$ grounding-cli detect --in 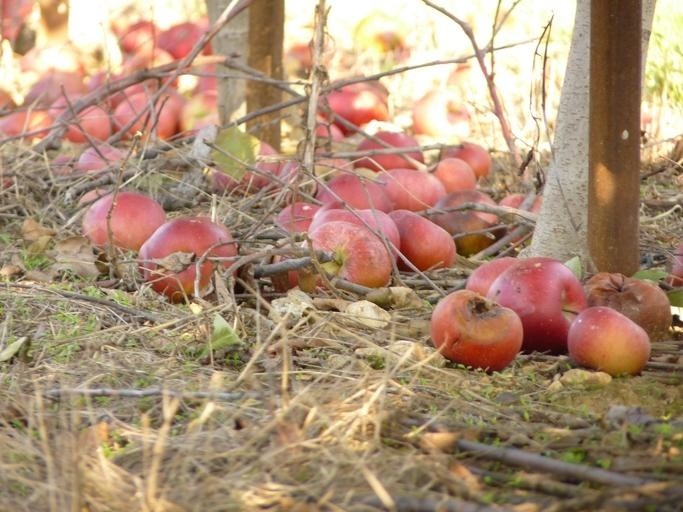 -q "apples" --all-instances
[1,1,673,378]
